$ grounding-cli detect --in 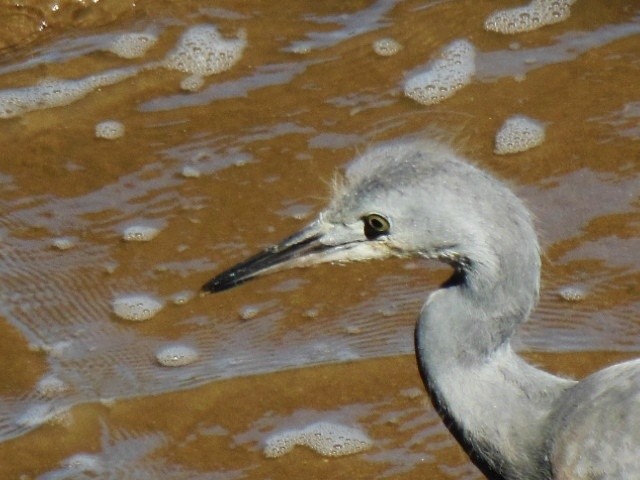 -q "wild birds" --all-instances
[197,133,640,480]
